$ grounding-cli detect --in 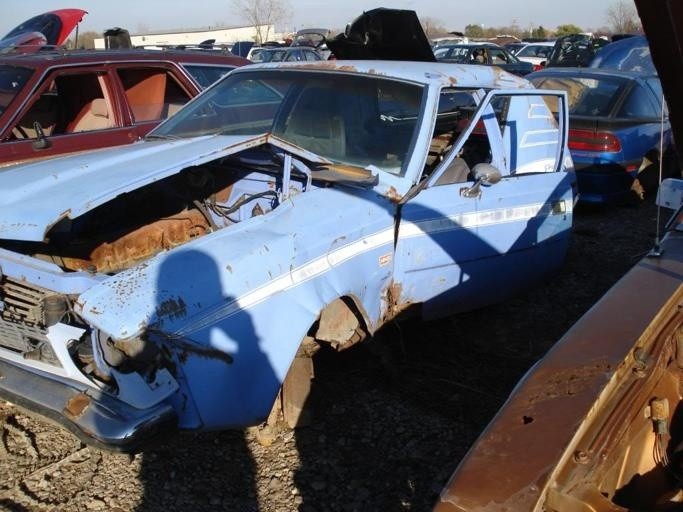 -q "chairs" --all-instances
[52,66,167,136]
[277,88,345,163]
